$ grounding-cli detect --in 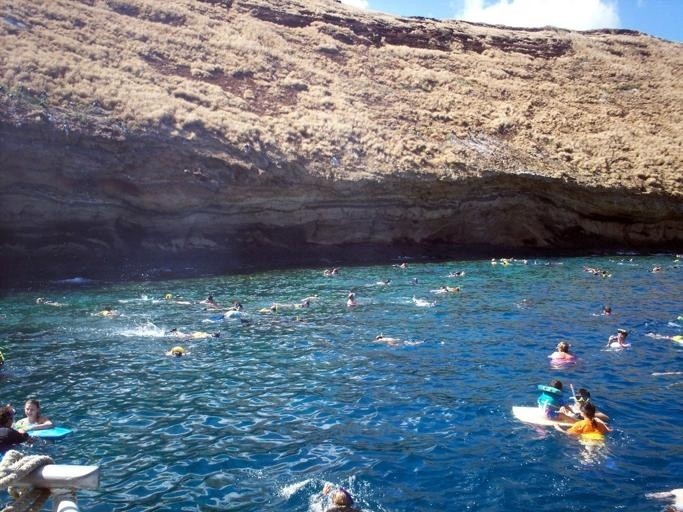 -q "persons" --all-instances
[16,398,53,431]
[540,377,564,419]
[553,401,613,438]
[314,481,361,512]
[647,330,683,346]
[604,305,612,315]
[548,342,576,362]
[1,405,32,449]
[607,329,630,348]
[562,388,611,423]
[582,252,682,279]
[35,252,529,361]
